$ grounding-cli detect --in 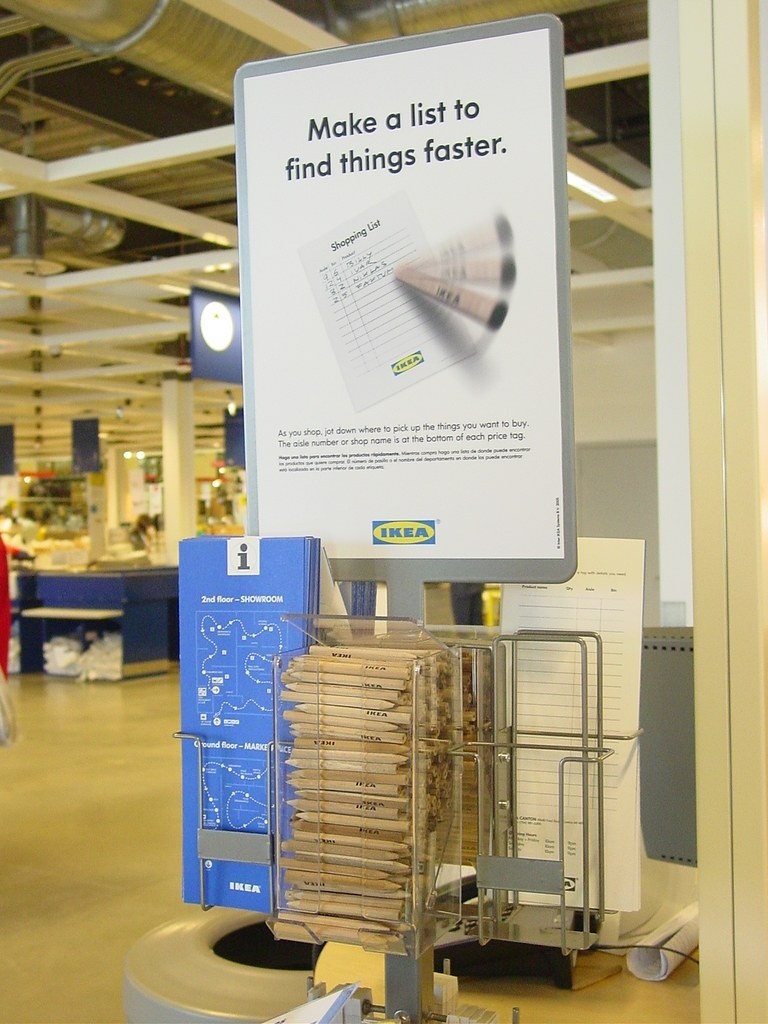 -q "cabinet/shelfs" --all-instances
[9,565,179,679]
